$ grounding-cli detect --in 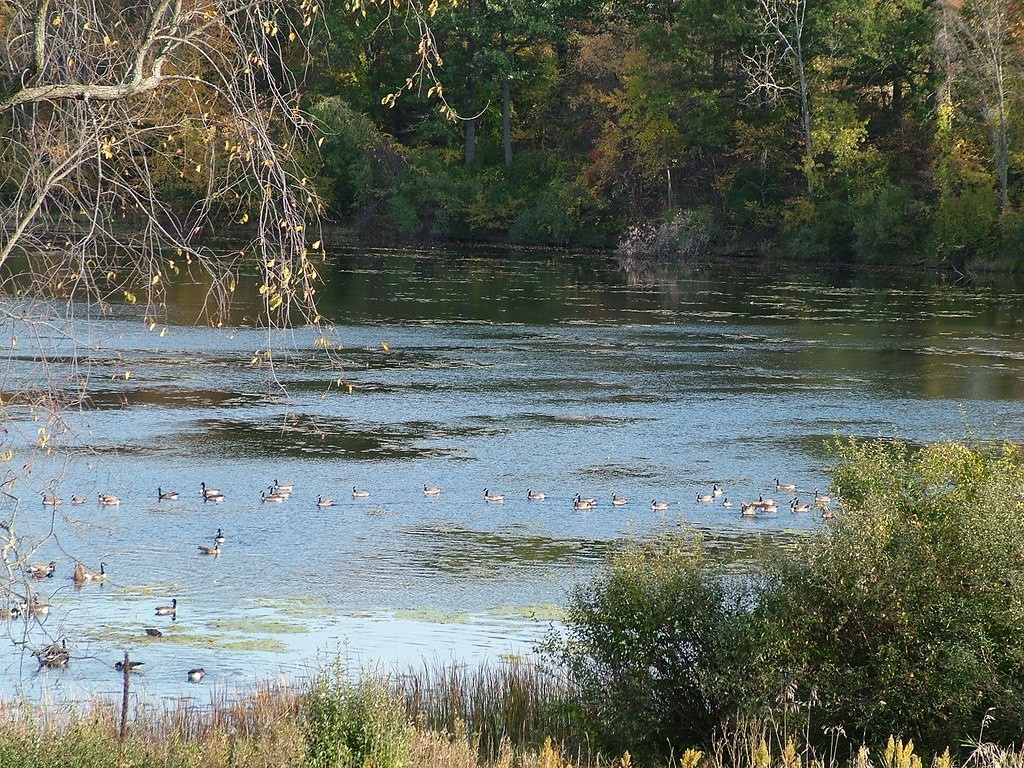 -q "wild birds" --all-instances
[0,477,837,683]
